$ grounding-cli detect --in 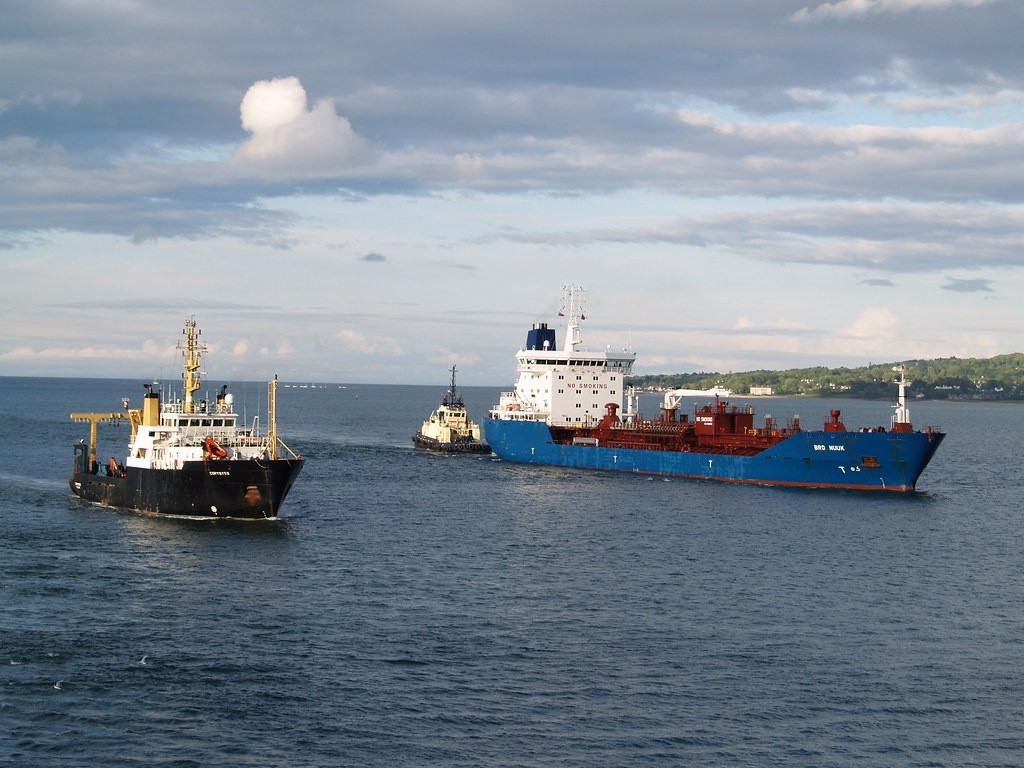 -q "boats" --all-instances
[66,312,309,524]
[671,385,733,398]
[412,366,494,455]
[481,286,949,493]
